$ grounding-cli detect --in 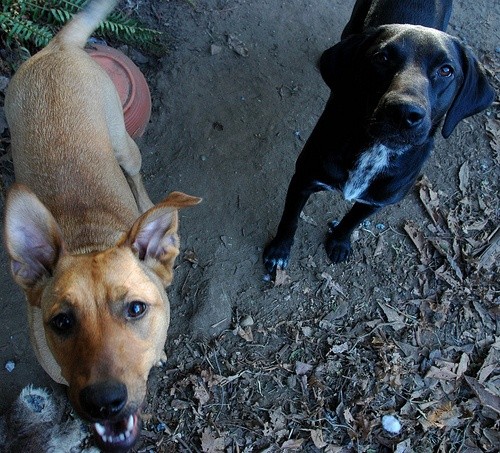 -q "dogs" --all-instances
[6,0,203,453]
[261,0,496,270]
